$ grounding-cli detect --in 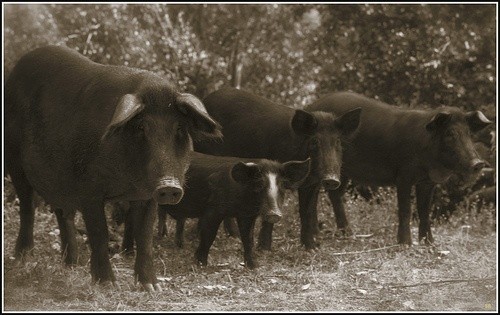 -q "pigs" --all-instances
[4,44,214,288]
[305,92,489,246]
[202,88,364,259]
[111,147,312,275]
